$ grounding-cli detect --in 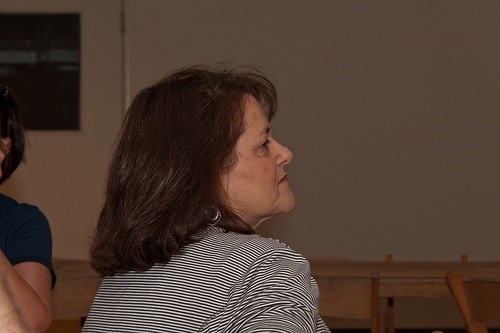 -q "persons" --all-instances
[0,80,56,333]
[82,65,331,333]
